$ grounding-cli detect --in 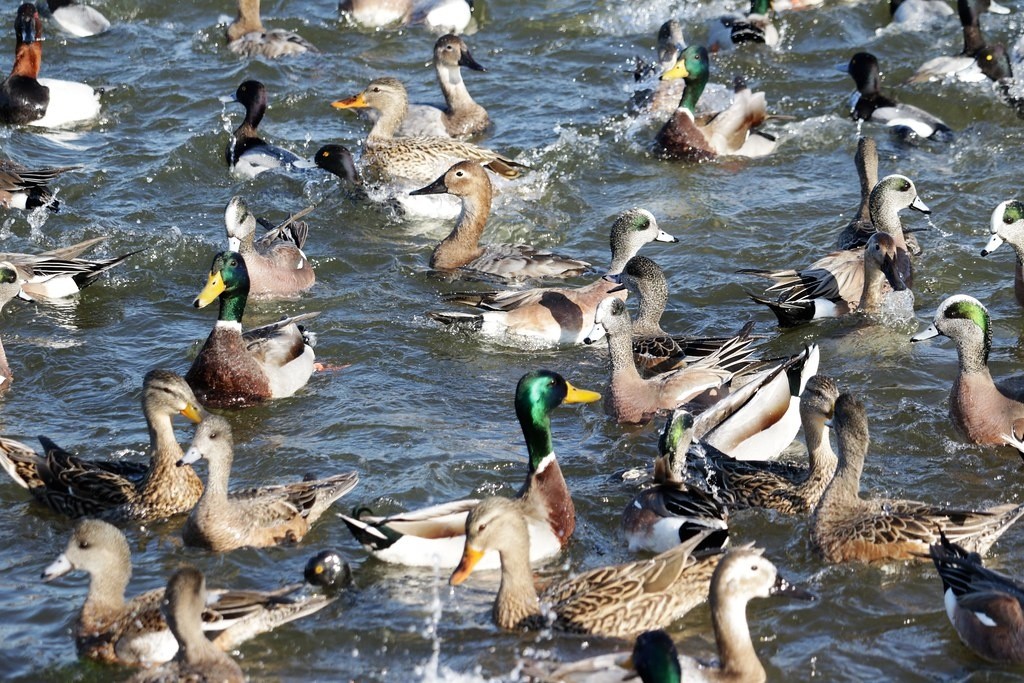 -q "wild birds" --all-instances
[0,0,1024,683]
